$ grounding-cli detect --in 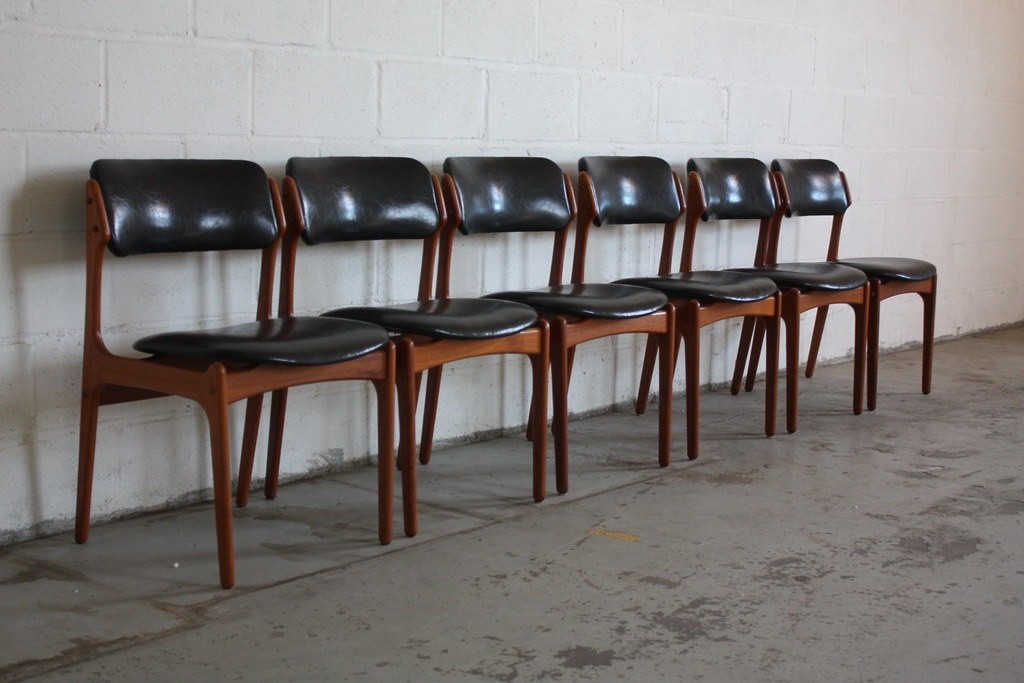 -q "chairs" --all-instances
[634,158,871,439]
[73,158,396,588]
[549,158,783,458]
[731,159,937,416]
[285,156,550,533]
[419,157,676,502]
